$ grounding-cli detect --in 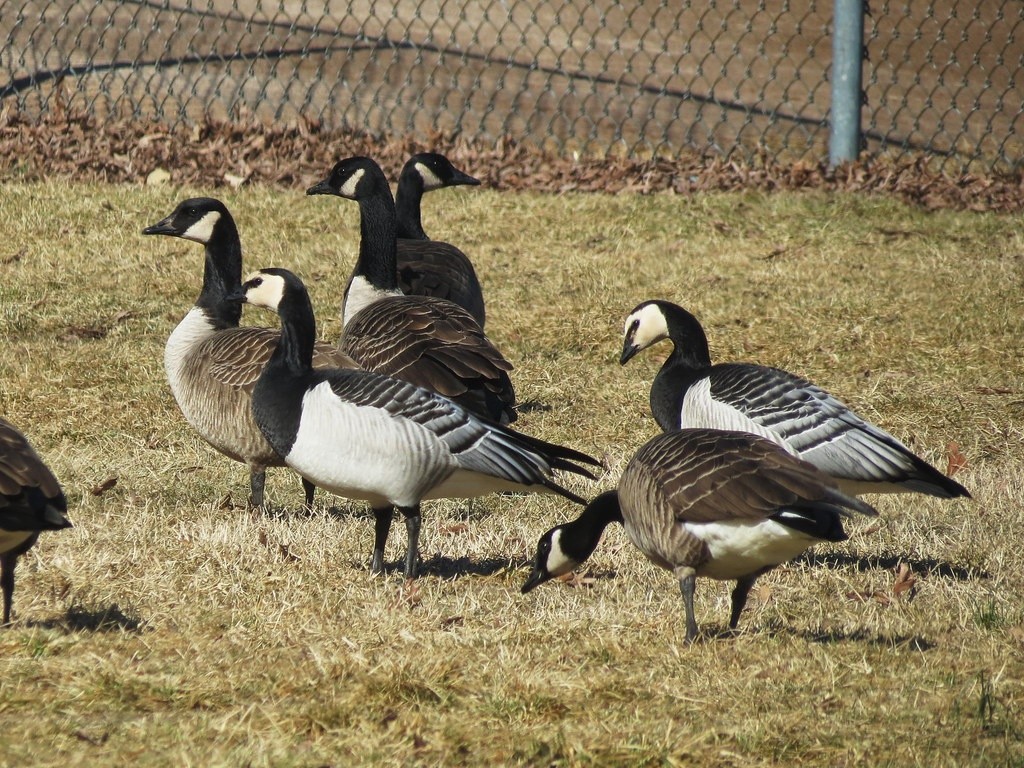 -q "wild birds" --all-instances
[0,149,976,647]
